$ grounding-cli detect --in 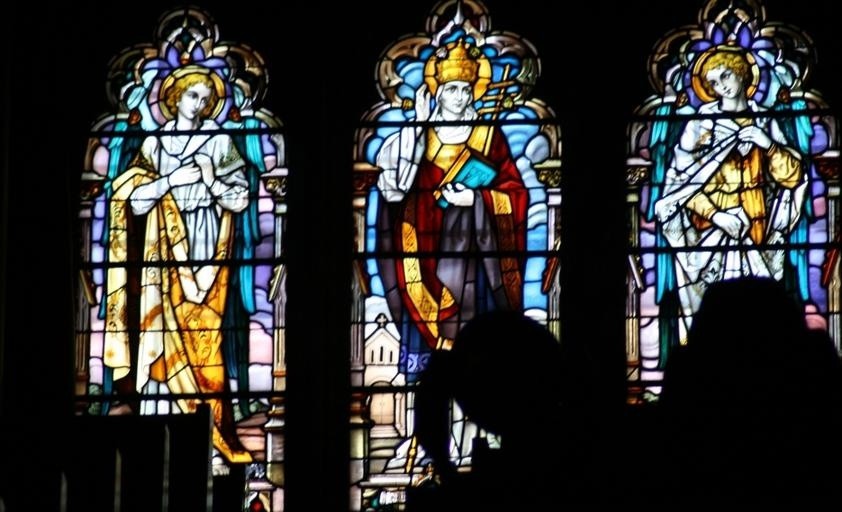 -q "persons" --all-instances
[651,45,815,371]
[377,80,529,474]
[413,275,842,512]
[106,72,257,477]
